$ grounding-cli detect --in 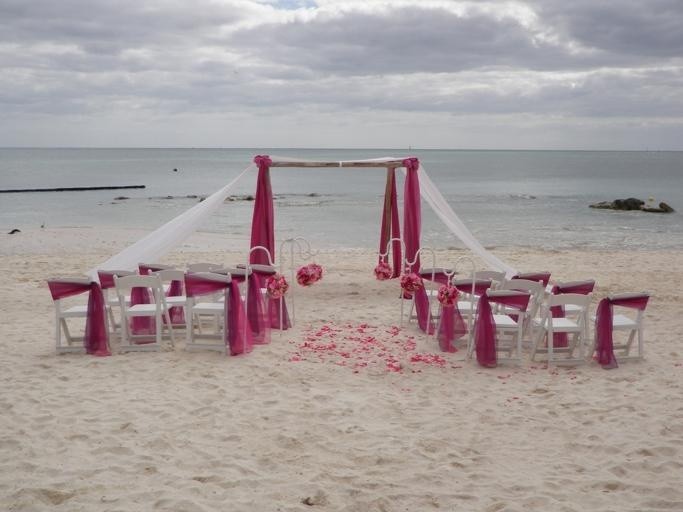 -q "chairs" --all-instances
[43,264,289,354]
[400,268,650,367]
